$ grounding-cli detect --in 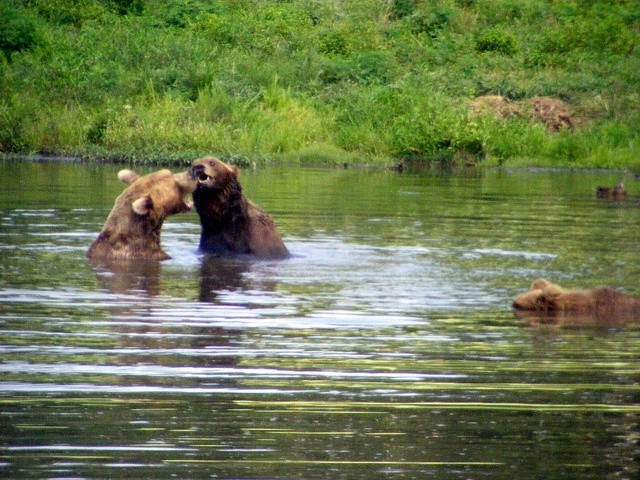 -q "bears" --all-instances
[190,155,299,262]
[512,278,640,311]
[85,156,199,267]
